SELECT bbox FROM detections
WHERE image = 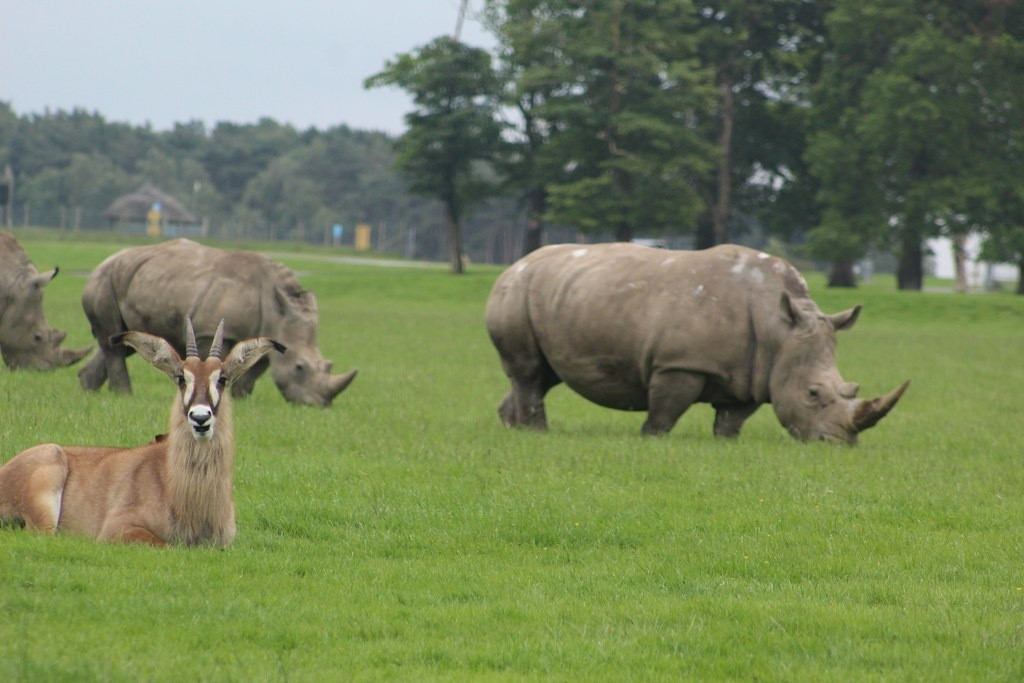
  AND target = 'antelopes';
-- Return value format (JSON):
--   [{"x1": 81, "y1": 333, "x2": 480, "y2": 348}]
[{"x1": 0, "y1": 312, "x2": 273, "y2": 552}]
